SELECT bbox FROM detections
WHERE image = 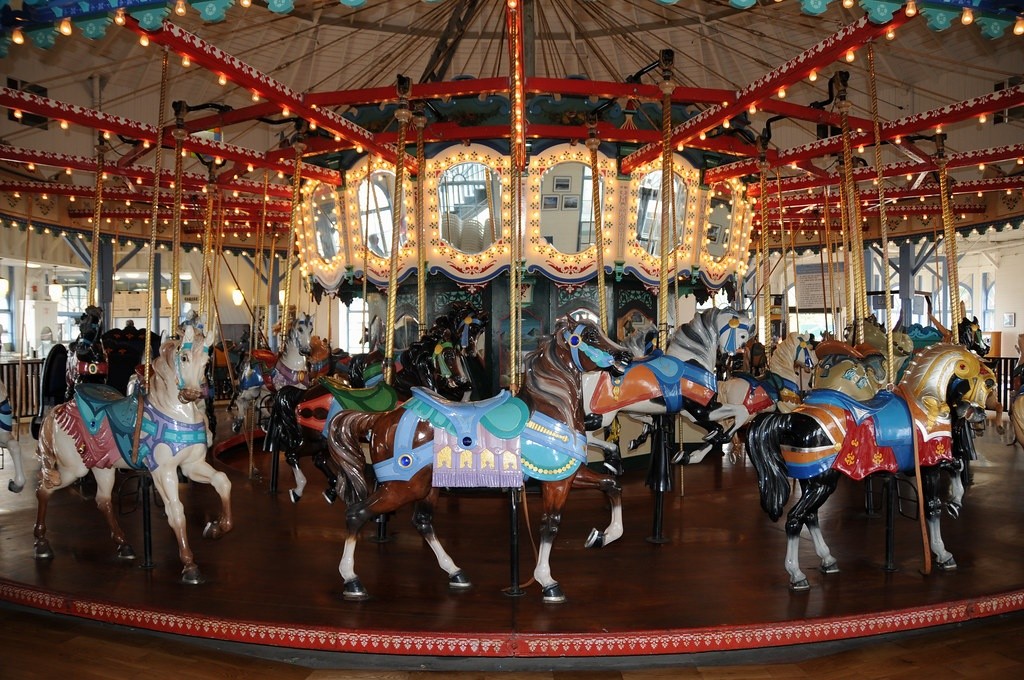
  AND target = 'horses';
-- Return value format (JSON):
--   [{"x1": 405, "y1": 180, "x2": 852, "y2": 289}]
[{"x1": 0, "y1": 303, "x2": 1017, "y2": 604}]
